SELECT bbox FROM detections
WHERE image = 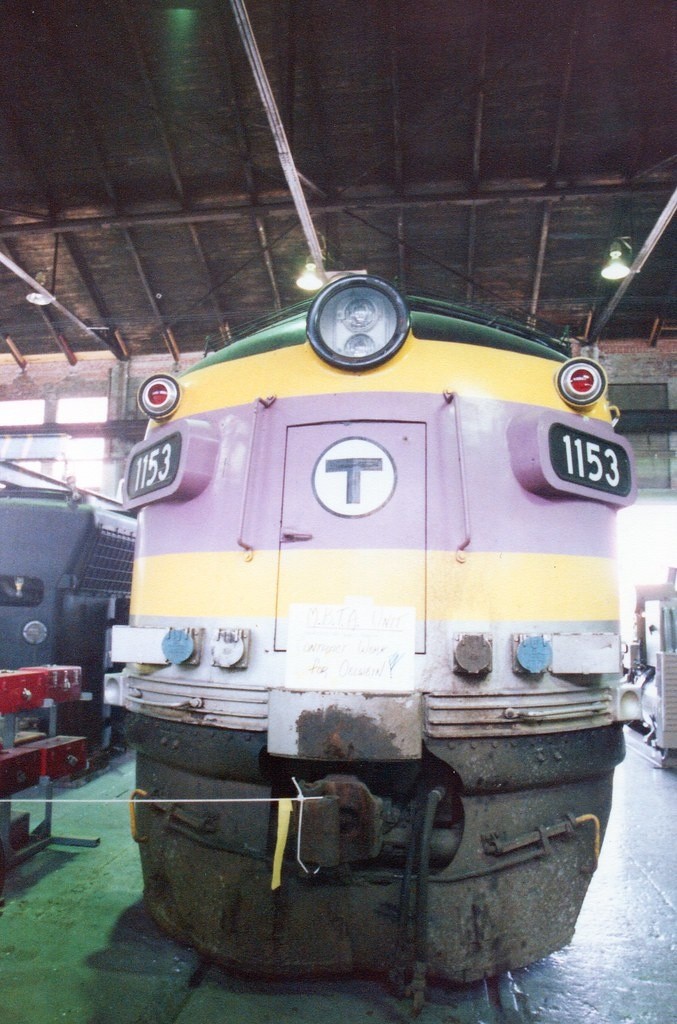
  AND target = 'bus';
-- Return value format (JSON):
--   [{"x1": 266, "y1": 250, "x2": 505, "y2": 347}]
[
  {"x1": 115, "y1": 273, "x2": 643, "y2": 1004},
  {"x1": 0, "y1": 480, "x2": 141, "y2": 774},
  {"x1": 617, "y1": 568, "x2": 677, "y2": 768}
]
